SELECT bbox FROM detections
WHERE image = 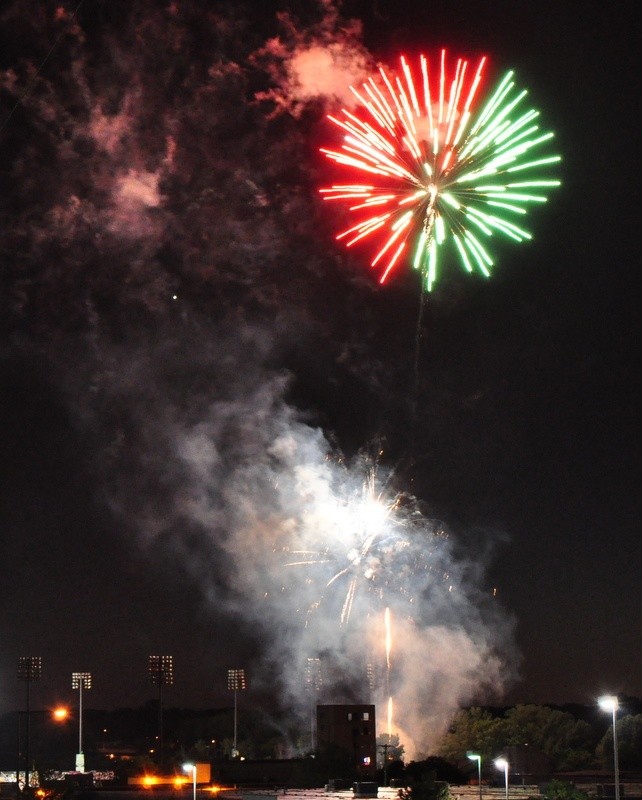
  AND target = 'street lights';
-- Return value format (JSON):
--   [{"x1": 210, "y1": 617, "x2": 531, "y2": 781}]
[
  {"x1": 72, "y1": 672, "x2": 91, "y2": 754},
  {"x1": 303, "y1": 657, "x2": 322, "y2": 754},
  {"x1": 467, "y1": 754, "x2": 482, "y2": 800},
  {"x1": 183, "y1": 764, "x2": 196, "y2": 800},
  {"x1": 228, "y1": 669, "x2": 245, "y2": 751},
  {"x1": 495, "y1": 757, "x2": 509, "y2": 800},
  {"x1": 598, "y1": 695, "x2": 621, "y2": 800},
  {"x1": 149, "y1": 655, "x2": 173, "y2": 769},
  {"x1": 17, "y1": 657, "x2": 42, "y2": 798}
]
